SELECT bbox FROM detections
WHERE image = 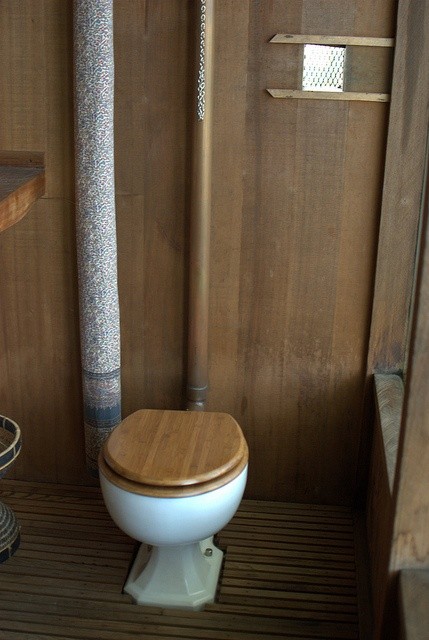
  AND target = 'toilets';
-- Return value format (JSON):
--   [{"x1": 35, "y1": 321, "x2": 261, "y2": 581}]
[{"x1": 96, "y1": 407, "x2": 251, "y2": 608}]
